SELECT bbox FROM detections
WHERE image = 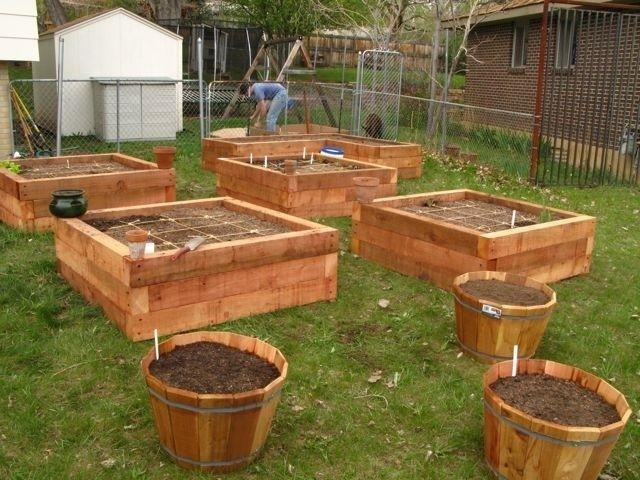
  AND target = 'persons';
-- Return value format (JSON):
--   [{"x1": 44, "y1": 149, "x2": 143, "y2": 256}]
[{"x1": 240, "y1": 82, "x2": 288, "y2": 134}]
